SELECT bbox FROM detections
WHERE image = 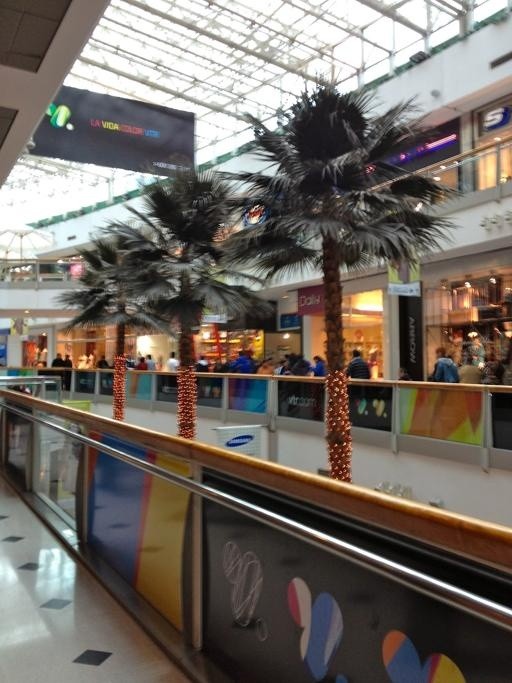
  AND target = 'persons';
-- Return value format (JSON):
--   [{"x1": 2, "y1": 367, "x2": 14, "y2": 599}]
[
  {"x1": 166, "y1": 352, "x2": 179, "y2": 371},
  {"x1": 97, "y1": 355, "x2": 109, "y2": 368},
  {"x1": 196, "y1": 350, "x2": 327, "y2": 376},
  {"x1": 52, "y1": 353, "x2": 72, "y2": 367},
  {"x1": 125, "y1": 353, "x2": 155, "y2": 371},
  {"x1": 430, "y1": 344, "x2": 505, "y2": 384},
  {"x1": 345, "y1": 349, "x2": 370, "y2": 379}
]
